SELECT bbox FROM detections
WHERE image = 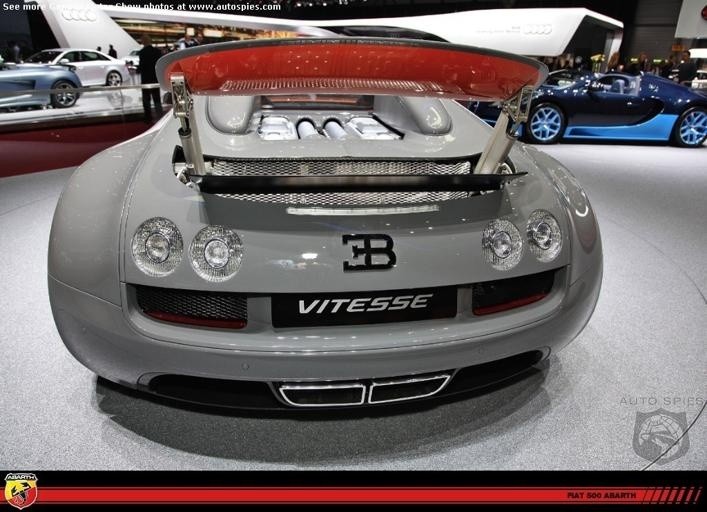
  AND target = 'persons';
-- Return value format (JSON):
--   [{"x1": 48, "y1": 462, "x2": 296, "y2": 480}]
[
  {"x1": 172, "y1": 33, "x2": 209, "y2": 51},
  {"x1": 108, "y1": 44, "x2": 117, "y2": 59},
  {"x1": 135, "y1": 34, "x2": 164, "y2": 124},
  {"x1": 536, "y1": 50, "x2": 697, "y2": 87}
]
